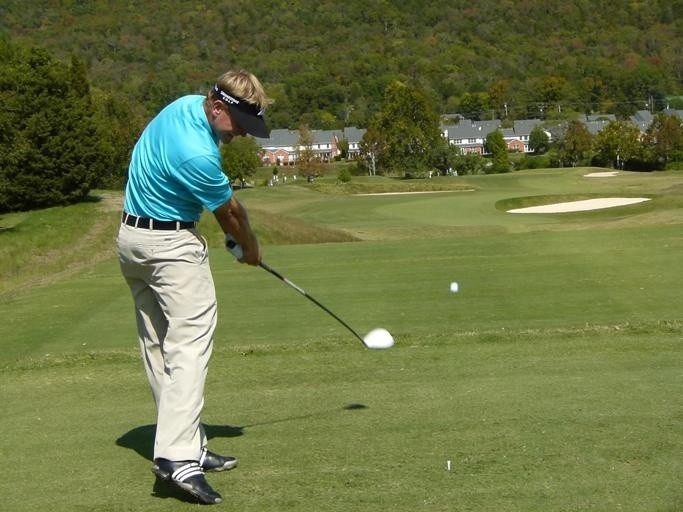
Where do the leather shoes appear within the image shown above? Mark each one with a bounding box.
[197,446,237,473]
[151,457,223,505]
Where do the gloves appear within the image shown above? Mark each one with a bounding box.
[222,234,264,267]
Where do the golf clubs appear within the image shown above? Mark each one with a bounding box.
[225,237,394,350]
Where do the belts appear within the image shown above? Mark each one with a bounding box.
[120,212,195,232]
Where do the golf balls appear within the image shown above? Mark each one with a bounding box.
[451,281,459,290]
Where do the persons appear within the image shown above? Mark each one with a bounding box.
[117,69,262,506]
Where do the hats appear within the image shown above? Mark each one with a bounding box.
[212,83,272,140]
